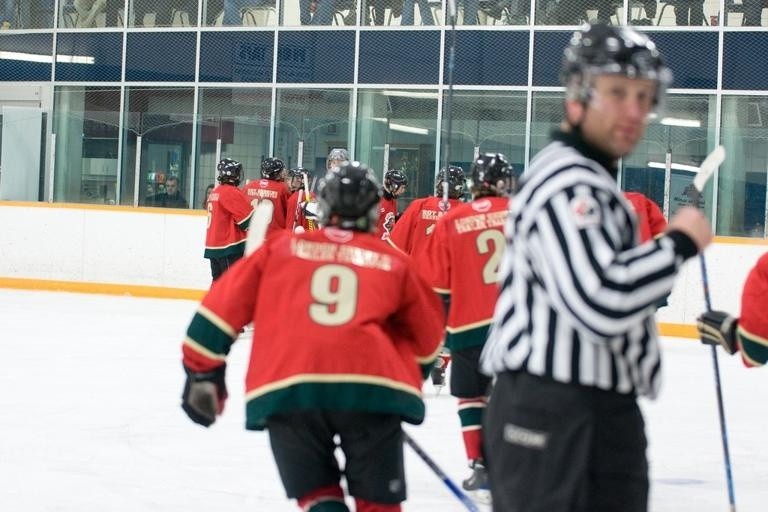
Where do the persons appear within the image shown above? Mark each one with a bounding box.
[420,150,513,490]
[479,19,713,512]
[388,164,467,386]
[240,149,352,258]
[377,171,406,241]
[695,250,767,369]
[202,184,214,209]
[0,0,767,28]
[178,160,445,512]
[145,175,189,207]
[623,191,666,244]
[203,160,255,334]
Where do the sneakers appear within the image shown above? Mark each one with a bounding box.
[432,368,446,384]
[463,467,488,489]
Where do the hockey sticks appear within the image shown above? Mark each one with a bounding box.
[241,199,481,512]
[690,145,737,512]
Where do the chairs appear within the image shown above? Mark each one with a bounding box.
[61,4,710,27]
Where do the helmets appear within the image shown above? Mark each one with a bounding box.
[436,154,515,198]
[563,24,670,83]
[218,157,308,191]
[316,148,408,218]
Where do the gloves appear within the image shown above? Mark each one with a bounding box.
[697,311,738,354]
[182,362,227,426]
